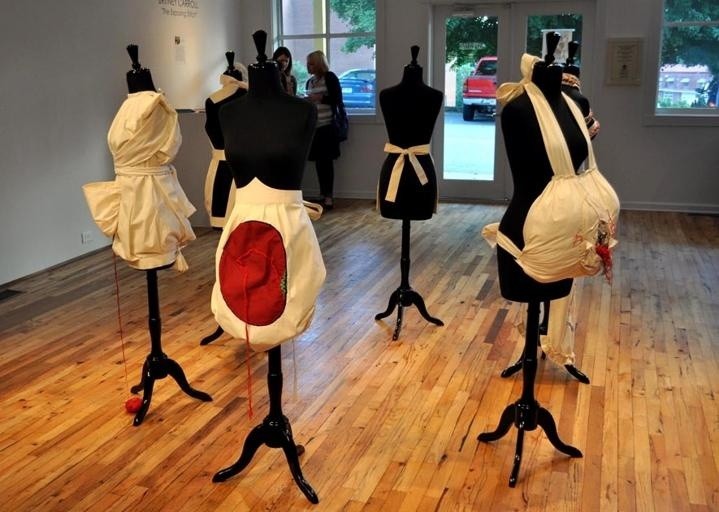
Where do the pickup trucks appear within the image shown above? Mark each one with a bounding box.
[462,58,497,120]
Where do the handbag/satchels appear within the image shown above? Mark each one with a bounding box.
[333,104,348,141]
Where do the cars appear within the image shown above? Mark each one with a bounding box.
[338,70,375,115]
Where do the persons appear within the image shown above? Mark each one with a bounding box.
[272,47,296,97]
[115,27,602,506]
[306,51,346,212]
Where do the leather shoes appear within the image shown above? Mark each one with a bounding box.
[325,200,334,210]
